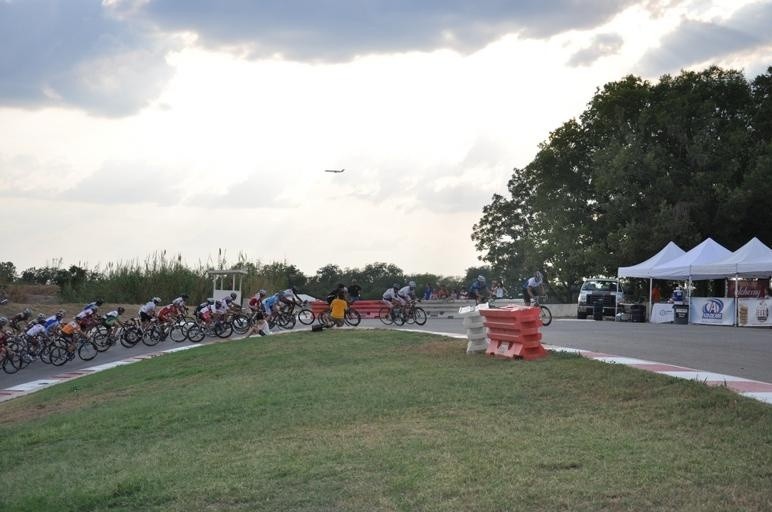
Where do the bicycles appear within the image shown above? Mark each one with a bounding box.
[468,293,497,308]
[380,299,417,325]
[391,299,427,326]
[518,292,553,326]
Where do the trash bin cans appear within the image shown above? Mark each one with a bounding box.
[593,300,604,321]
[631,305,646,322]
[672,304,689,324]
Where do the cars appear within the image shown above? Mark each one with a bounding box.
[578,279,626,319]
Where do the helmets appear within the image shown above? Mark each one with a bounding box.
[408,281,416,290]
[533,271,544,281]
[207,293,237,307]
[279,290,285,296]
[292,285,300,292]
[478,275,485,282]
[153,293,189,308]
[392,282,401,290]
[260,290,266,296]
[0,298,125,327]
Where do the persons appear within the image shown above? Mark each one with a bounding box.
[326,279,362,326]
[0,286,303,356]
[652,283,661,303]
[672,286,683,302]
[382,274,546,311]
